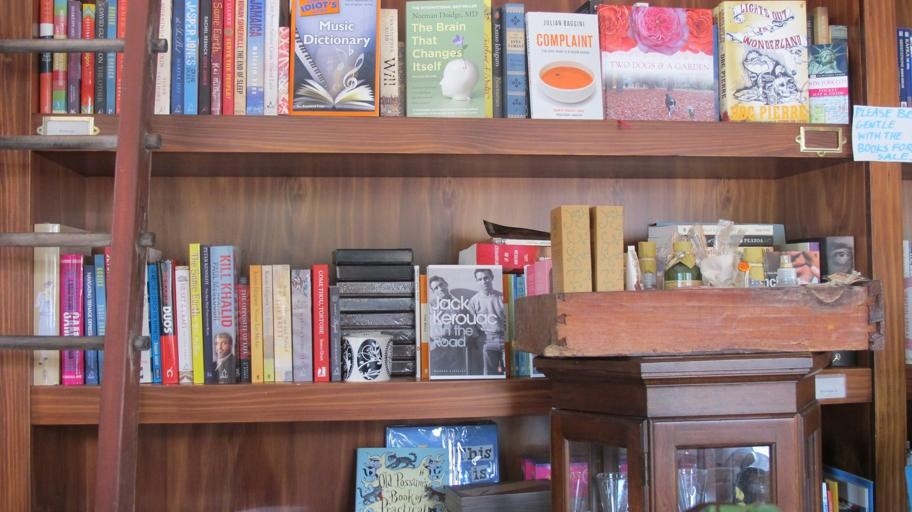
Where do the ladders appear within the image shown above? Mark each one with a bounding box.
[0,0,167,512]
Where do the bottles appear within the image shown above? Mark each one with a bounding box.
[664,235,702,287]
[732,261,749,287]
[638,241,656,288]
[776,254,797,287]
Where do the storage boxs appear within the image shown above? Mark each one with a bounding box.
[508,280,887,358]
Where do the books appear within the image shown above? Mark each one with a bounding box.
[406,1,850,124]
[820,462,874,512]
[898,28,911,107]
[34,0,405,117]
[458,223,854,378]
[34,223,507,385]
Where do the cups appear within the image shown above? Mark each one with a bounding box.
[343,333,393,384]
[677,466,708,508]
[595,471,626,512]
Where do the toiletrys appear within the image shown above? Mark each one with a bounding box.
[624,244,643,291]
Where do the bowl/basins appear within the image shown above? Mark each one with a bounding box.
[536,59,597,105]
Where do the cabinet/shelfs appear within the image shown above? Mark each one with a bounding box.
[529,348,839,512]
[0,0,887,512]
[887,1,912,511]
